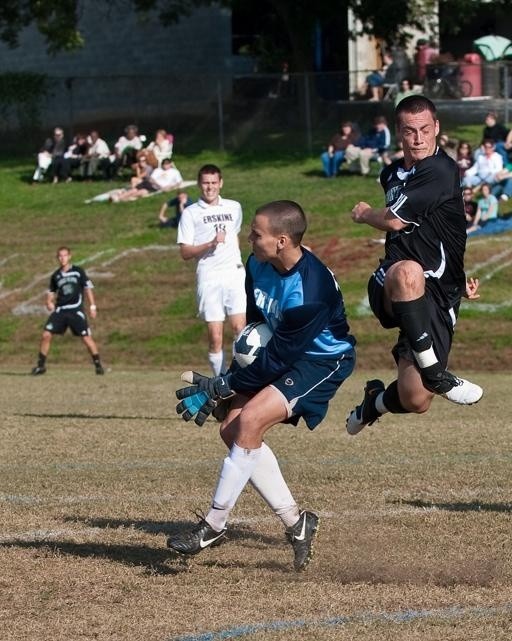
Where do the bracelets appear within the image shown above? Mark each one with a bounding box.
[90,304,96,311]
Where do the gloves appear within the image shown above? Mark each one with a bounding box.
[175,359,244,425]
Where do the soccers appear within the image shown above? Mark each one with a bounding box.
[233,321,273,371]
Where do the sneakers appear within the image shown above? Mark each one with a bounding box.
[422,368,483,405]
[285,509,320,573]
[167,506,228,554]
[95,366,103,375]
[32,367,45,375]
[346,379,385,435]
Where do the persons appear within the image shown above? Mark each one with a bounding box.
[343,93,485,436]
[176,163,248,378]
[165,199,358,574]
[27,121,184,204]
[30,245,104,375]
[319,33,512,235]
[157,190,193,229]
